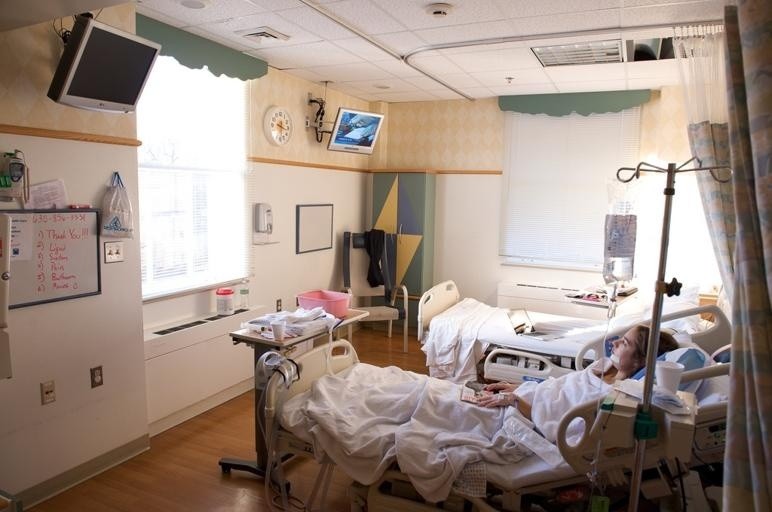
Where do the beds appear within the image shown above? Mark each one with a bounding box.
[261,342,731,512]
[415,280,730,387]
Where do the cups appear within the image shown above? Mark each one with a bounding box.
[654,360,685,394]
[270,320,286,341]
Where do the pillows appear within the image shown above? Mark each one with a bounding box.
[609,302,699,335]
[629,349,712,395]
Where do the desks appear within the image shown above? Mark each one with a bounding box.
[572,285,637,309]
[219,308,370,493]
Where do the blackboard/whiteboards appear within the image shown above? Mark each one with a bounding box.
[0,208,102,311]
[296,204,334,254]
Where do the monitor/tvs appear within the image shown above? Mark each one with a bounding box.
[327,107,384,155]
[47,14,162,114]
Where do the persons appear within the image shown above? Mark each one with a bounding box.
[344,114,379,139]
[476,325,680,443]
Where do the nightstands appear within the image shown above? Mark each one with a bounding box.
[699,294,718,323]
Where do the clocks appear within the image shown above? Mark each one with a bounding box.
[264,105,292,147]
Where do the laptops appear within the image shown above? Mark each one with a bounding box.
[506,307,564,342]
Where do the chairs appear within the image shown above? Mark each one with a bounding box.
[336,232,408,352]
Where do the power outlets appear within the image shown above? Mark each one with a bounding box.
[104,241,124,263]
[90,366,103,388]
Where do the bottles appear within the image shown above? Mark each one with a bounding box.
[216,288,235,316]
[347,288,354,309]
[239,279,249,310]
